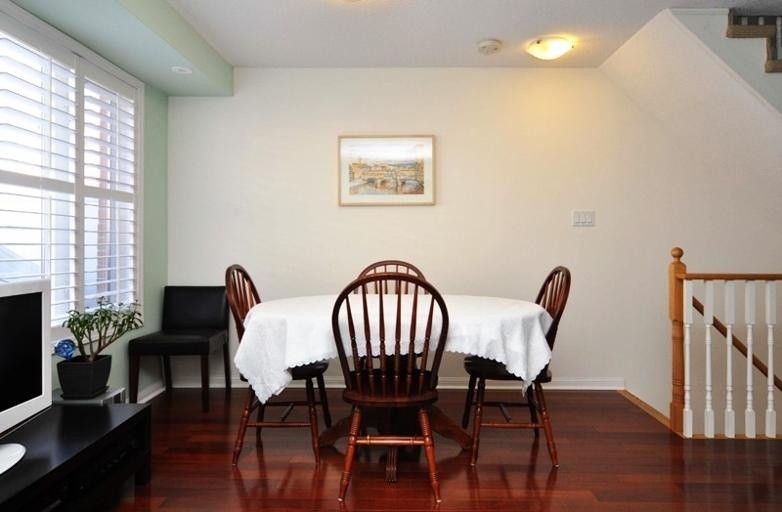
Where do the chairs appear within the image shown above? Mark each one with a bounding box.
[353,260,429,295]
[224,264,331,466]
[331,272,449,503]
[127,286,233,415]
[462,265,570,466]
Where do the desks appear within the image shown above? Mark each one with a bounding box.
[0,402,151,510]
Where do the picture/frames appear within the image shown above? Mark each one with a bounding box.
[334,131,436,208]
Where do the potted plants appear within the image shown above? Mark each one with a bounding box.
[50,298,143,400]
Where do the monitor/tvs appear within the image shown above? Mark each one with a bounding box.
[0,276,52,475]
